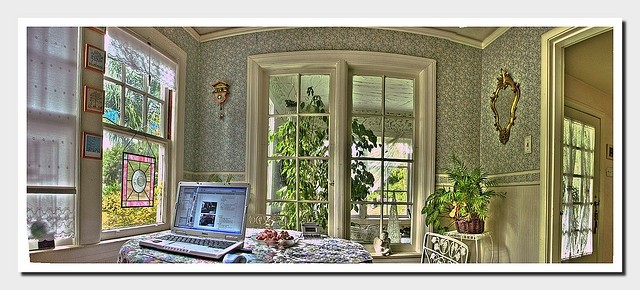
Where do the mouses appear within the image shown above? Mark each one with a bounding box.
[221,253,246,263]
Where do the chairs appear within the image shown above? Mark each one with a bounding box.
[420,231,471,263]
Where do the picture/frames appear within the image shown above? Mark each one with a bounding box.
[88,27,107,36]
[81,131,103,159]
[84,42,106,73]
[83,84,105,115]
[606,143,613,159]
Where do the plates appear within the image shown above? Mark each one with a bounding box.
[251,233,300,247]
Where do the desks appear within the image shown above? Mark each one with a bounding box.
[122,227,373,263]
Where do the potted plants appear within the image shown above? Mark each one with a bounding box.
[421,153,507,236]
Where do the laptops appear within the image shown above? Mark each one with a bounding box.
[139,181,252,259]
